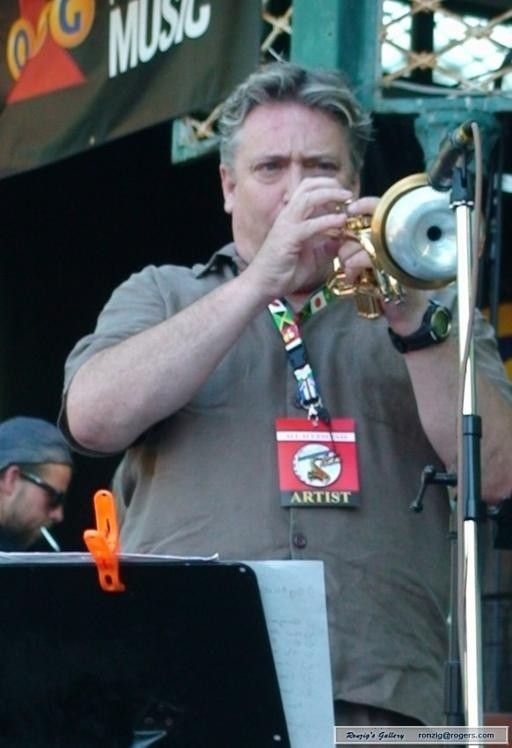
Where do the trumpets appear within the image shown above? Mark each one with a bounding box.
[320,174,486,319]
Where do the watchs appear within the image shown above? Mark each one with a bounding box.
[387,297,453,354]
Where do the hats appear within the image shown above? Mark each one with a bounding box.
[0,414,74,472]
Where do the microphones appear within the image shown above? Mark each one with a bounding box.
[426,121,473,190]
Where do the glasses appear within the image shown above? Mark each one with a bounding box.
[21,474,68,510]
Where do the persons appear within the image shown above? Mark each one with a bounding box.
[56,63,511,748]
[1,415,90,552]
